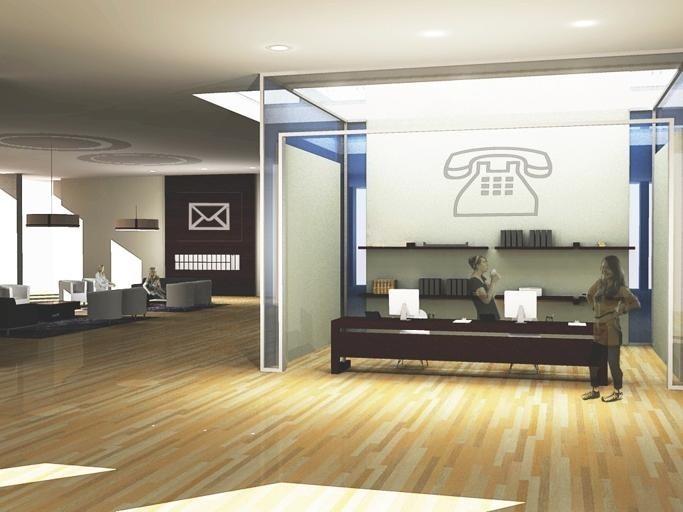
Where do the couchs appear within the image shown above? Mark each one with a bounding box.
[130,277,193,306]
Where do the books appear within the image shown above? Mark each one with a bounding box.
[418,278,472,297]
[371,278,397,296]
[499,229,552,248]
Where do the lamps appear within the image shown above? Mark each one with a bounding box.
[25,145,80,228]
[112,205,160,233]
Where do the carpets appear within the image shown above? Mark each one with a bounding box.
[5,315,160,338]
[145,301,230,313]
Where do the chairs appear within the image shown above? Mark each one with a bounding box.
[58,277,114,307]
[166,279,213,311]
[85,287,149,325]
[509,304,543,373]
[0,298,38,335]
[397,308,428,369]
[0,284,30,306]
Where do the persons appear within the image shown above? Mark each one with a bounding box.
[93,263,116,291]
[467,255,502,320]
[580,255,642,403]
[141,267,165,300]
